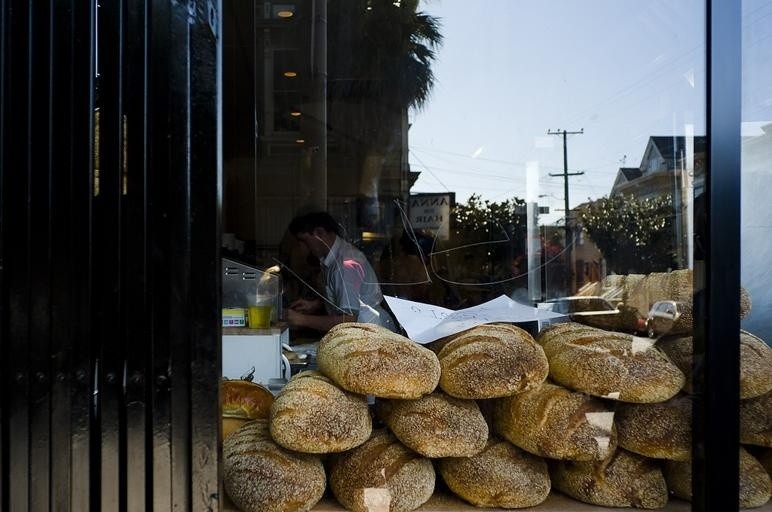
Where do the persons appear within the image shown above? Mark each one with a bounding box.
[278,209,402,339]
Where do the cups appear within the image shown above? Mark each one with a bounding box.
[246,293,273,330]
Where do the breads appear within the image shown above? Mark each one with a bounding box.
[222,268,772,511]
